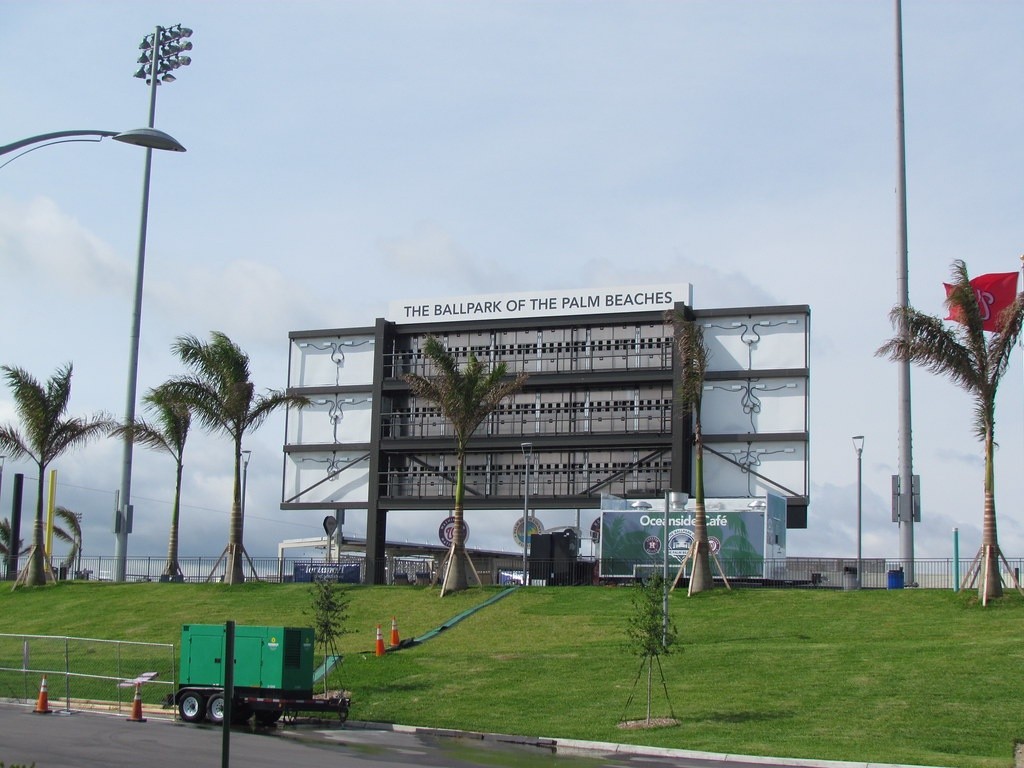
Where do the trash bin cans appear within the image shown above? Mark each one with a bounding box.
[887,569,904,589]
[843,566,858,589]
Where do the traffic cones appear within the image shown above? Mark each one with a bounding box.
[127,684,147,722]
[33,675,53,713]
[388,615,399,647]
[375,623,386,657]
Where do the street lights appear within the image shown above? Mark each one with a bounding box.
[852,434,866,591]
[521,441,533,588]
[112,24,194,587]
[0,454,6,498]
[240,448,252,547]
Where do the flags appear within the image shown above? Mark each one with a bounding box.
[942,271,1020,333]
